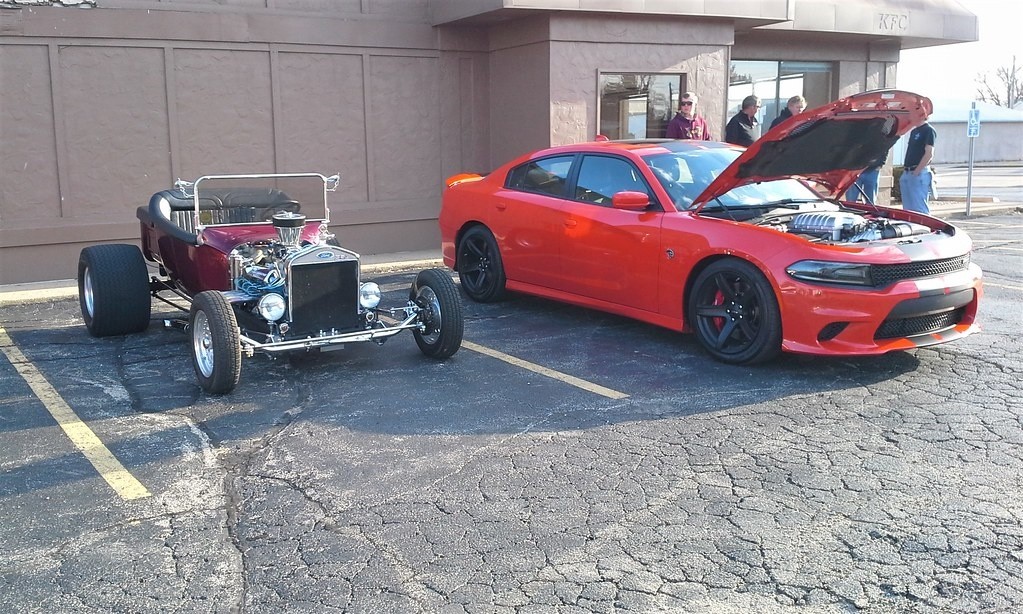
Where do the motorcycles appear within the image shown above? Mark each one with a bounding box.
[77,172,463,395]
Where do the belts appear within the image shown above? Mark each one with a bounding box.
[904,166,916,170]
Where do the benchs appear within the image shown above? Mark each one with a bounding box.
[150,187,304,234]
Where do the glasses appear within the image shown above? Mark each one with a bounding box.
[679,100,693,106]
[755,105,759,108]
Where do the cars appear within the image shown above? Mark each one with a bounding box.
[438,88,983,366]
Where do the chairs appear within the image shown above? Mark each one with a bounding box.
[653,158,680,182]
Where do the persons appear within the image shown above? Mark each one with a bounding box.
[846,148,889,203]
[770,96,807,129]
[898,116,937,215]
[666,92,711,140]
[726,95,761,147]
